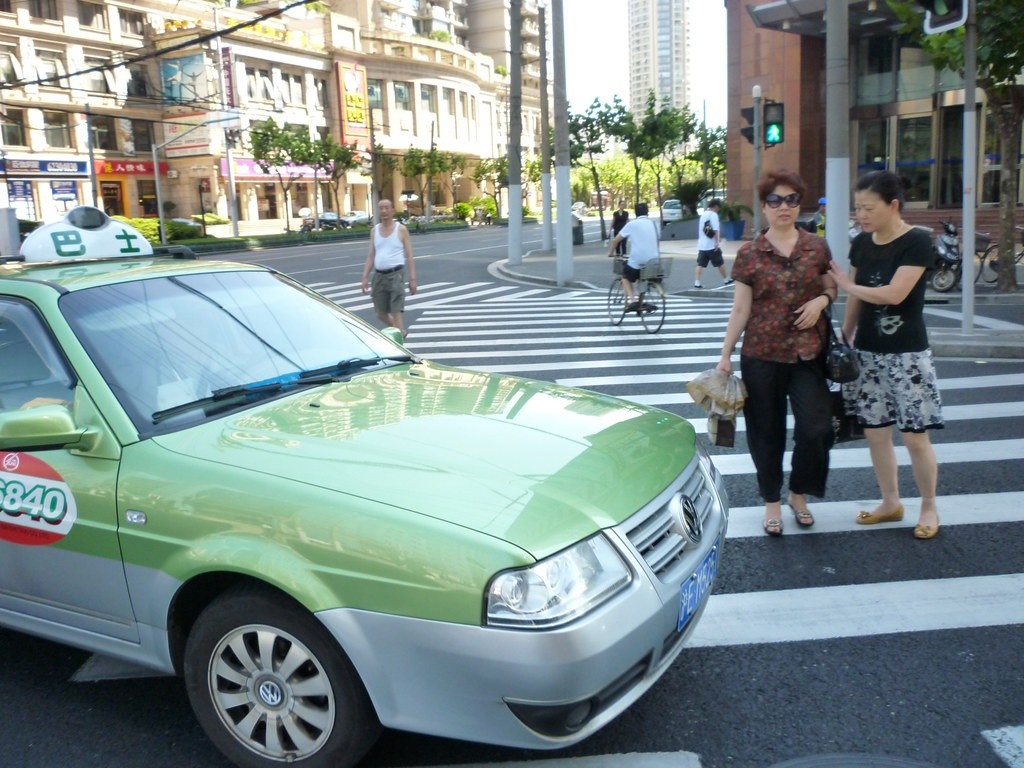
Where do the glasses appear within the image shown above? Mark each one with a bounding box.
[765,193,802,208]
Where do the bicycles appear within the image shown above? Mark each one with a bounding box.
[954,231,992,293]
[981,225,1024,284]
[608,254,672,333]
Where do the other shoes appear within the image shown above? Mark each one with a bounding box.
[637,311,640,317]
[694,285,705,289]
[724,279,735,284]
[856,505,904,524]
[913,514,938,538]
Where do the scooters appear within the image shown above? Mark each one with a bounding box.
[925,219,962,293]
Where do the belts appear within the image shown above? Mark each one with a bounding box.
[376,265,403,274]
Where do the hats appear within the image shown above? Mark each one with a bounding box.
[818,198,826,205]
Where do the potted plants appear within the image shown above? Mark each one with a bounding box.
[710,200,753,241]
[164,201,176,216]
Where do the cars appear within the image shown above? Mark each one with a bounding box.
[1,245,728,768]
[660,199,690,226]
[340,211,368,228]
[303,213,349,231]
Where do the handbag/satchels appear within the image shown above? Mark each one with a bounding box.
[648,268,664,288]
[826,385,866,444]
[703,221,715,238]
[604,236,611,248]
[814,293,860,383]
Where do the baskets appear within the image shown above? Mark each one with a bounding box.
[613,259,625,274]
[975,235,992,252]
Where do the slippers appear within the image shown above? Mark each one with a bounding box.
[789,502,814,527]
[763,519,784,535]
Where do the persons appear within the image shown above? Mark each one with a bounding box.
[477,209,483,226]
[695,199,735,288]
[813,197,825,237]
[716,167,838,538]
[828,170,944,538]
[362,200,416,336]
[609,203,660,316]
[608,201,629,255]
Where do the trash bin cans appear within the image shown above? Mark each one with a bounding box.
[572,220,583,245]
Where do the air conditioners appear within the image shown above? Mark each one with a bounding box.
[168,171,179,179]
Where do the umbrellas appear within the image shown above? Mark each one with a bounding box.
[399,194,419,202]
[298,208,311,216]
[686,368,747,418]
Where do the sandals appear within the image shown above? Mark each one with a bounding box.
[624,302,640,312]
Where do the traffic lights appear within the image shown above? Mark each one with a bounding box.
[912,0,969,35]
[739,107,753,145]
[763,103,784,143]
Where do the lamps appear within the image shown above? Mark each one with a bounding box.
[783,20,791,31]
[868,0,877,13]
